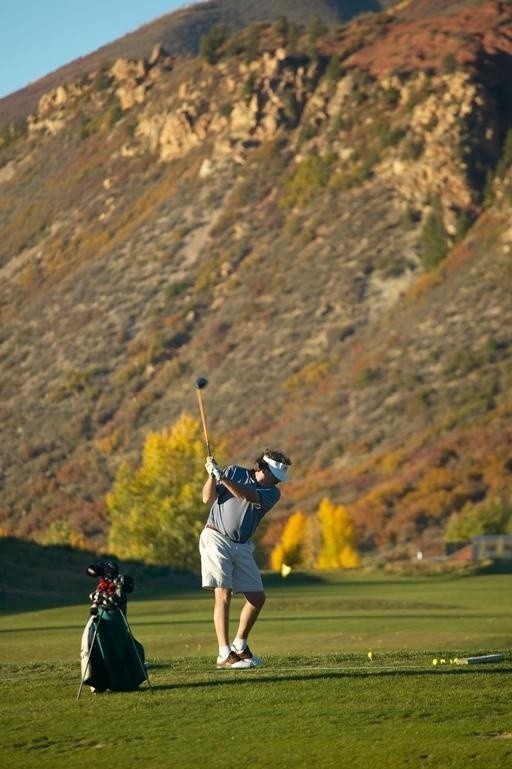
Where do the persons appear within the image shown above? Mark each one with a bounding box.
[195,446,293,668]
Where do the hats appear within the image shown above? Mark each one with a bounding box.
[263,455,290,482]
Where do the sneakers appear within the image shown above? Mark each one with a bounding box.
[216,644,260,669]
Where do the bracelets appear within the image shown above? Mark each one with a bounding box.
[211,464,226,481]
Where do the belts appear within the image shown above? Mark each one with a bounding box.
[207,525,222,535]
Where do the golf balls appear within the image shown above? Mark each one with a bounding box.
[433,657,459,665]
[368,651,375,657]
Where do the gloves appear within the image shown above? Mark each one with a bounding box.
[204,462,225,482]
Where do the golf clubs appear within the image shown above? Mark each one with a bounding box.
[86,561,133,594]
[195,377,211,458]
